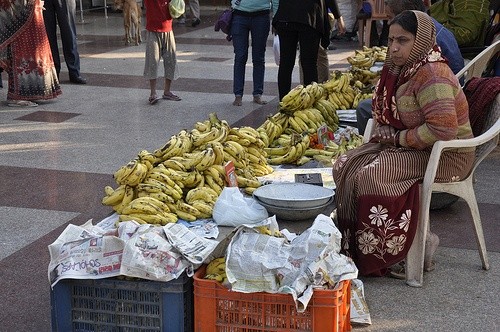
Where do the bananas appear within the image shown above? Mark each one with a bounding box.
[101,112,272,228]
[255,46,388,166]
[202,225,282,280]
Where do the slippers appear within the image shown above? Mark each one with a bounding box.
[390,266,405,279]
[7,100,38,107]
[162,91,182,101]
[149,95,160,104]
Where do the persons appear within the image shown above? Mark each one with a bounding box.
[143,0,182,103]
[333,11,476,277]
[176,0,201,26]
[356,0,465,137]
[0,0,87,106]
[228,0,344,106]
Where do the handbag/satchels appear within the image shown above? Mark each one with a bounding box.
[215,9,232,35]
[273,35,280,65]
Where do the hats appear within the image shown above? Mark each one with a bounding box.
[168,0,185,18]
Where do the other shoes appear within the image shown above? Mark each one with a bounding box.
[69,76,88,84]
